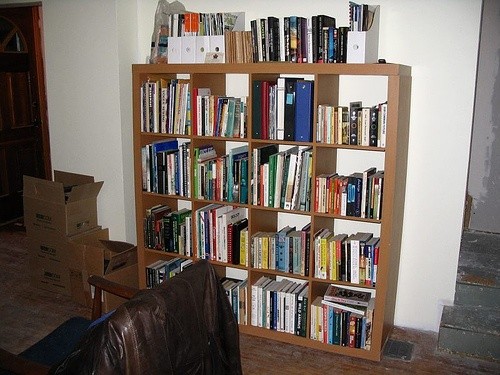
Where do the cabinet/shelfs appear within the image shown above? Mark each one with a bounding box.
[130,63,413,362]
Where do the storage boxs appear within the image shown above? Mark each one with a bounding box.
[23,169,140,321]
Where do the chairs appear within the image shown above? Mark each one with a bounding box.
[0,259,209,375]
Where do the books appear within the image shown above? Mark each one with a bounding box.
[140,2,387,352]
